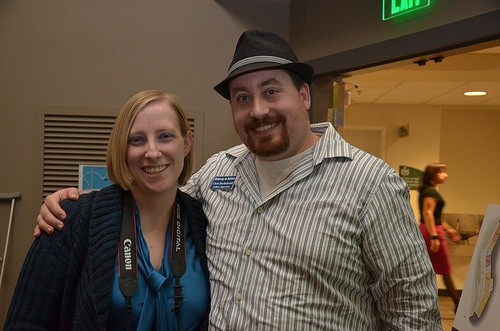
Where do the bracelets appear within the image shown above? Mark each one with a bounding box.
[431,236,439,240]
[444,226,453,231]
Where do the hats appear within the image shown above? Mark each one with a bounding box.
[213,30,315,100]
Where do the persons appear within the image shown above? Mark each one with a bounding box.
[419,163,462,314]
[4,90,211,331]
[34,30,443,331]
